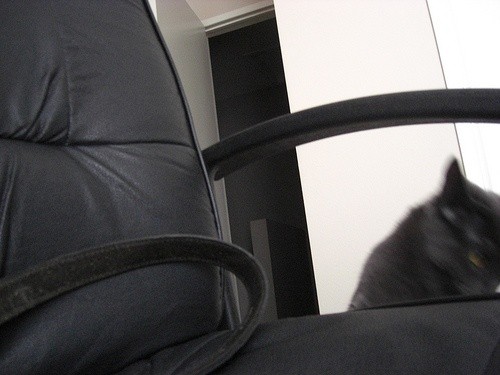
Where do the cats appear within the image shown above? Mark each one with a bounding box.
[348,156,500,311]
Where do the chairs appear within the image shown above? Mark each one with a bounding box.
[0,0,499,375]
